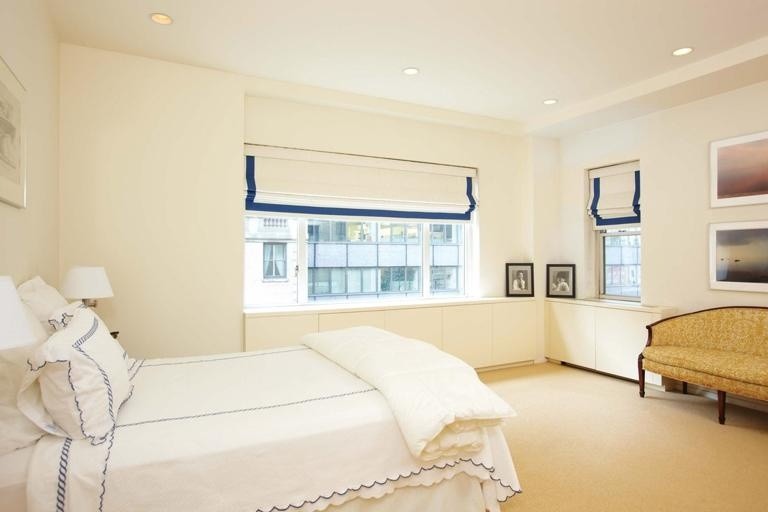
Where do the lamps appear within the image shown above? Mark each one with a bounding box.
[60,266,114,312]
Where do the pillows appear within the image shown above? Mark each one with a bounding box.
[0,272,137,454]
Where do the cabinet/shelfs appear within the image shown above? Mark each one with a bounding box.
[544,301,677,392]
[245,298,540,377]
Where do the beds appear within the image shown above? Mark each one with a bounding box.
[0,324,498,512]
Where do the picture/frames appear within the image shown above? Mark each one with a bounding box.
[546,264,576,298]
[706,129,768,294]
[505,262,534,297]
[0,55,30,213]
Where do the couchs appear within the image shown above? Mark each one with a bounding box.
[637,305,767,425]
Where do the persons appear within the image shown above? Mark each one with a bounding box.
[513,271,525,290]
[551,271,569,292]
